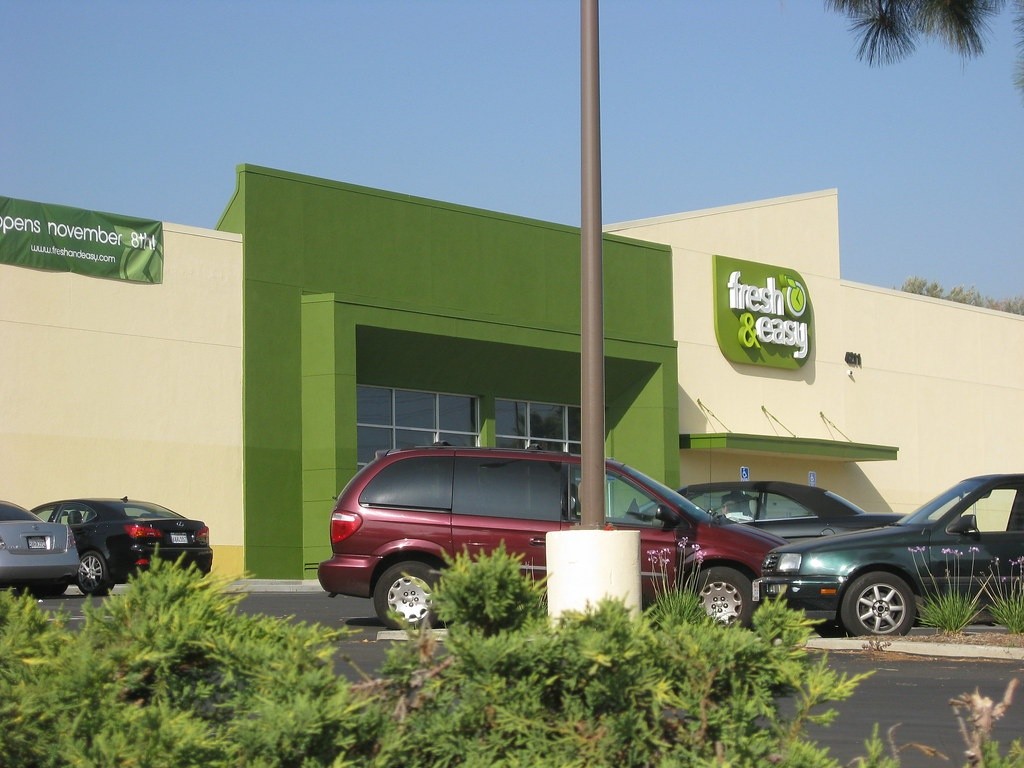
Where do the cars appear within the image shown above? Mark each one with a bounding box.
[631,473,1024,637]
[1,495,212,596]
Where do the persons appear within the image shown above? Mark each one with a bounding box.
[720,494,756,523]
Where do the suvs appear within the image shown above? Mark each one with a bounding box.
[315,440,794,635]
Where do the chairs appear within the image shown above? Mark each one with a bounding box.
[67,511,82,524]
